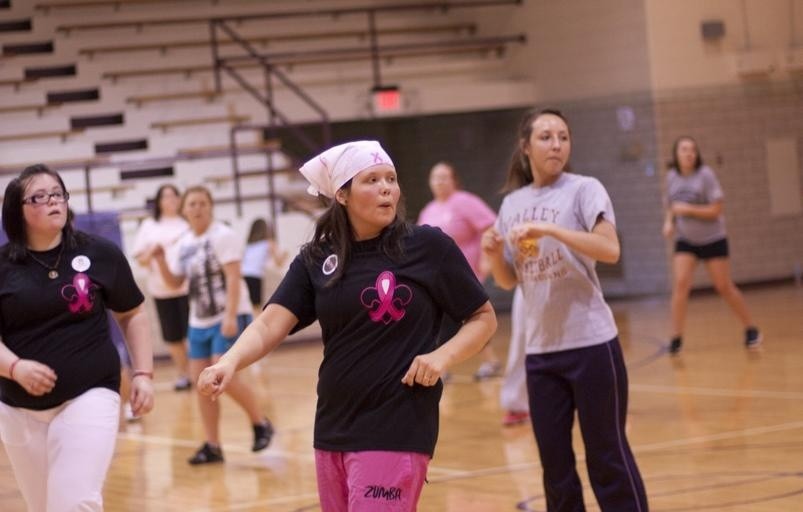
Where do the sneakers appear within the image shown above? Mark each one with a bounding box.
[501,411,528,426]
[743,324,764,349]
[174,376,191,391]
[189,441,224,465]
[473,359,505,380]
[669,335,685,355]
[252,415,275,452]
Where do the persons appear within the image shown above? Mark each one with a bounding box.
[413,160,501,381]
[239,212,289,312]
[129,183,194,391]
[661,133,766,355]
[139,183,275,466]
[195,138,499,512]
[0,162,156,511]
[480,105,650,511]
[500,284,530,426]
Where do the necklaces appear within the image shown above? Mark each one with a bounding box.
[26,240,65,281]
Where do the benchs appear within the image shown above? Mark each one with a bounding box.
[0,1,509,358]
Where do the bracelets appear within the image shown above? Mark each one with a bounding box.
[8,356,23,382]
[131,370,154,381]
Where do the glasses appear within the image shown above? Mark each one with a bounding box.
[21,191,70,206]
